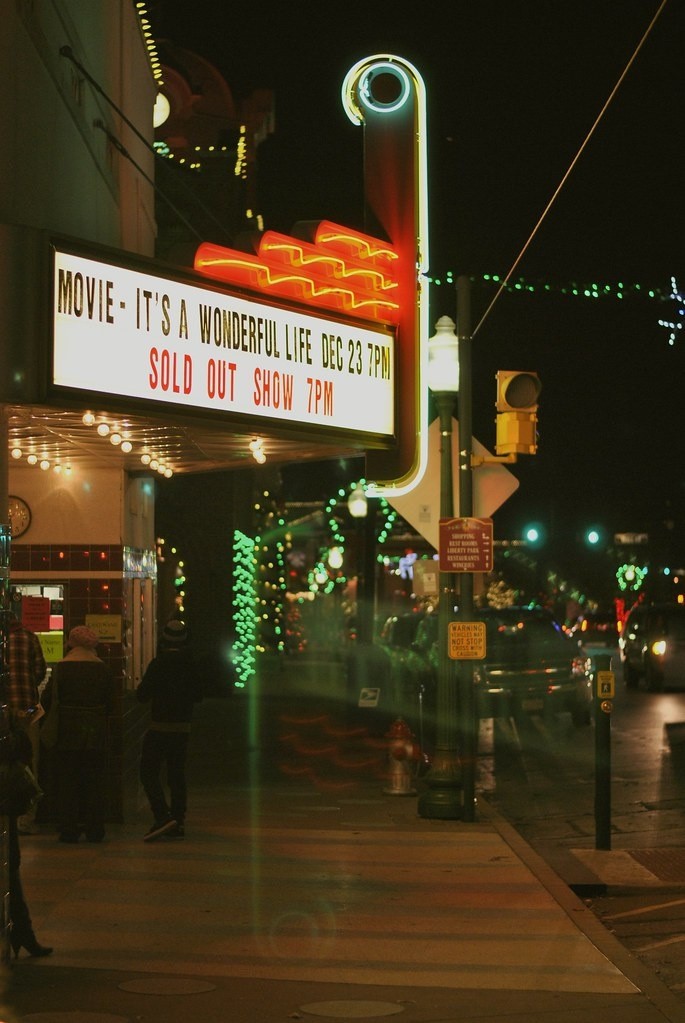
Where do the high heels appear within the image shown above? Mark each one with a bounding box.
[9,930,54,959]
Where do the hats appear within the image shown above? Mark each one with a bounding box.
[0,592,21,621]
[69,626,99,648]
[156,620,192,652]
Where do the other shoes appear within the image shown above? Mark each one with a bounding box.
[157,825,185,841]
[143,812,177,841]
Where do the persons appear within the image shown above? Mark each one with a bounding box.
[0,609,47,837]
[0,709,54,957]
[135,620,205,844]
[41,626,115,844]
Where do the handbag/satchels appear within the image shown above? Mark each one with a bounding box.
[0,700,44,814]
[40,667,58,750]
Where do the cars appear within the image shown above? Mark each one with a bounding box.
[620,599,685,693]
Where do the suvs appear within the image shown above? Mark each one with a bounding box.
[473,606,594,730]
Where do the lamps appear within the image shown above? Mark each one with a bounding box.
[141,454,150,465]
[110,433,122,446]
[64,467,72,475]
[28,454,38,465]
[248,438,267,464]
[53,464,62,474]
[150,459,159,470]
[82,414,95,426]
[120,440,133,453]
[164,468,173,478]
[11,449,22,459]
[158,464,166,474]
[97,423,110,437]
[40,460,50,471]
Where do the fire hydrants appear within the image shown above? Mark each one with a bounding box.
[369,721,431,796]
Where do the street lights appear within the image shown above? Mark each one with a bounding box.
[416,315,480,819]
[345,480,374,725]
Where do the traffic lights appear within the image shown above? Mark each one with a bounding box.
[496,371,542,412]
